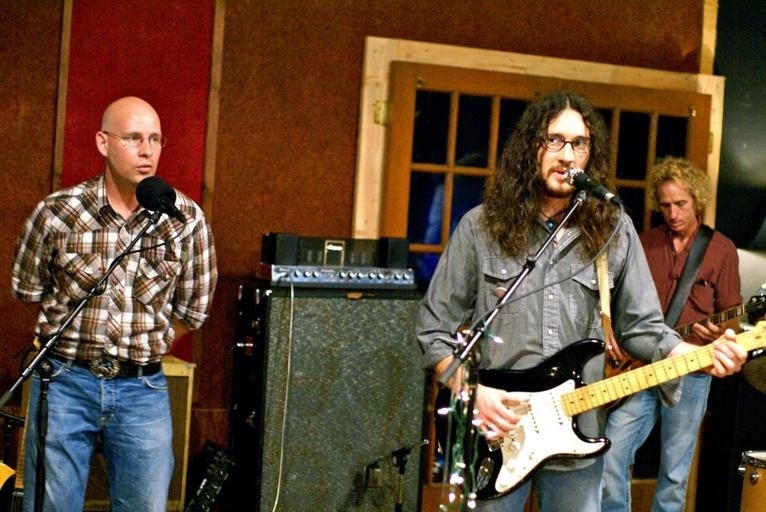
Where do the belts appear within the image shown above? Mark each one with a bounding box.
[47,355,163,380]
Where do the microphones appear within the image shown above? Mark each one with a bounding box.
[565,168,623,204]
[135,175,188,223]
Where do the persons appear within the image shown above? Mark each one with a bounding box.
[413,89,748,512]
[10,96,220,511]
[598,156,743,512]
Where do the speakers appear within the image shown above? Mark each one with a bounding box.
[229,285,428,510]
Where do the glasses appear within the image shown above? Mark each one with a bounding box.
[542,134,592,153]
[103,131,167,151]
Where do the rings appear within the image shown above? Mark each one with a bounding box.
[489,422,496,431]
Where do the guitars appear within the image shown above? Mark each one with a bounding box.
[435,319,766,500]
[600,283,766,412]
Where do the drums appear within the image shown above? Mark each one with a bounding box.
[737,449,766,511]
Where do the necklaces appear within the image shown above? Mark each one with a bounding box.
[537,209,568,230]
